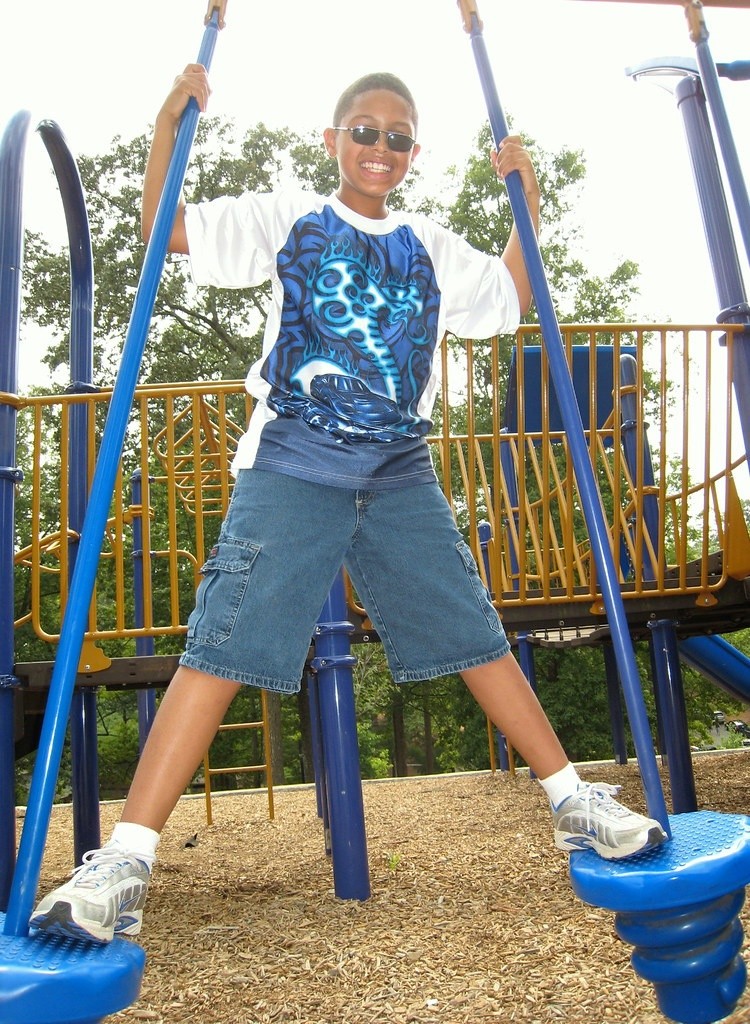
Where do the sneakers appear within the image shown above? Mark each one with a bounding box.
[27,839,149,944]
[551,782,668,861]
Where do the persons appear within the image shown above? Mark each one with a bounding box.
[30,62,672,944]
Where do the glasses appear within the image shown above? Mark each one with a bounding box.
[334,126,416,153]
